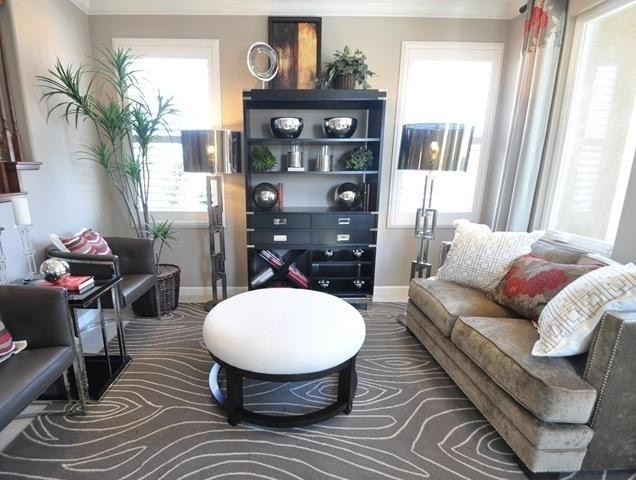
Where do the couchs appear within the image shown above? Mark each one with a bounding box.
[407,235,636,478]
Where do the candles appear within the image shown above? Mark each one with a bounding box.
[10,197,32,228]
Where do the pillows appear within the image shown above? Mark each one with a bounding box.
[0,317,19,362]
[435,219,546,294]
[47,227,113,258]
[530,263,636,357]
[494,254,605,320]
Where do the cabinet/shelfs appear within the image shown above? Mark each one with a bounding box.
[242,89,386,312]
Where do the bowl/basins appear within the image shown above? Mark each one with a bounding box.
[321,117,358,138]
[270,117,303,139]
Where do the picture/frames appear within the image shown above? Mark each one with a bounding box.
[267,15,324,91]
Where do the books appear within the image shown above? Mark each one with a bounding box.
[250,266,273,289]
[67,282,94,295]
[287,264,310,289]
[260,249,285,268]
[31,275,95,290]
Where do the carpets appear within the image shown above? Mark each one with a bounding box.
[0,302,549,479]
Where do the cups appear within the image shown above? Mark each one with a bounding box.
[286,144,304,168]
[316,144,333,172]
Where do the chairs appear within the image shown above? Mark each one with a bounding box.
[0,287,85,435]
[44,237,161,361]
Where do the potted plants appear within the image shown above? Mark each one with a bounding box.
[315,46,379,90]
[34,42,179,317]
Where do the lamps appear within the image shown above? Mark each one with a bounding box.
[183,129,242,311]
[397,123,474,280]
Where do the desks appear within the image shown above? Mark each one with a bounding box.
[11,274,136,407]
